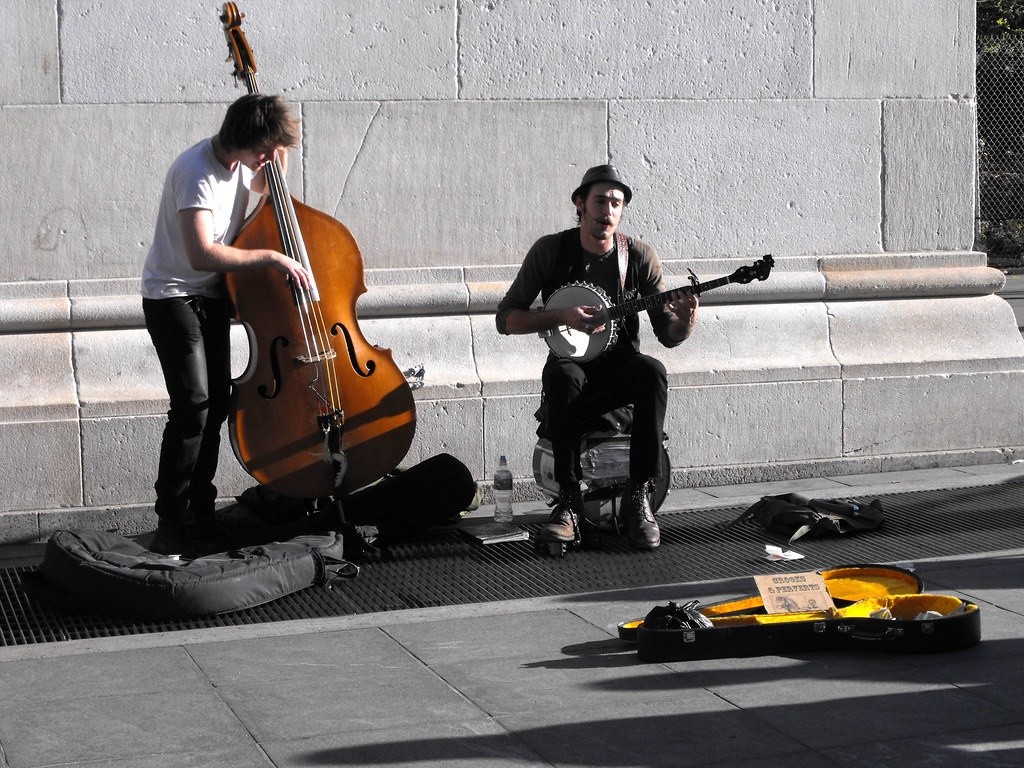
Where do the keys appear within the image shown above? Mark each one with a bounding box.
[184,295,206,318]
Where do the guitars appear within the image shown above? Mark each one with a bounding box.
[540,254,775,364]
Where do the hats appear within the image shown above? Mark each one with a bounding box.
[571,165,632,206]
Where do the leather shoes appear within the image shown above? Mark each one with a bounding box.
[149,507,230,555]
[619,477,660,548]
[535,487,585,542]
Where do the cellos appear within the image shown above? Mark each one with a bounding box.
[220,3,417,523]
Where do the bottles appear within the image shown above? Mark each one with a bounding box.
[494,455,514,523]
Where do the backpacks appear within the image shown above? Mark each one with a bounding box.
[716,492,884,546]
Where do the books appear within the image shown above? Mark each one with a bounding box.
[456,517,530,545]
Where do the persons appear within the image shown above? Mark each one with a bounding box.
[495,165,700,548]
[141,93,315,558]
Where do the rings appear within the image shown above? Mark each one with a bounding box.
[584,324,591,332]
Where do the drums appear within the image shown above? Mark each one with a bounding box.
[533,406,671,529]
